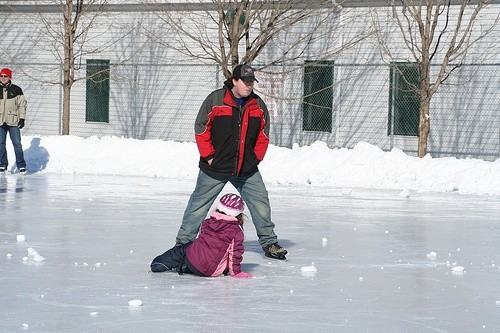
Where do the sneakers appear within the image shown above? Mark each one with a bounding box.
[261,243,287,260]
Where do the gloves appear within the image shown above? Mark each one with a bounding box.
[18,119,25,128]
[234,271,253,278]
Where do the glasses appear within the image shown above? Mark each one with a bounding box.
[1,75,9,77]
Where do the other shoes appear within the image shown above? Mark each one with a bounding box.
[20,168,27,174]
[0,166,8,174]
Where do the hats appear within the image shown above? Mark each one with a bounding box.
[215,192,245,216]
[0,68,12,77]
[233,64,258,83]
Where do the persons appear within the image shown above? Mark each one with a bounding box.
[0,68,27,173]
[174,64,288,260]
[151,193,252,278]
